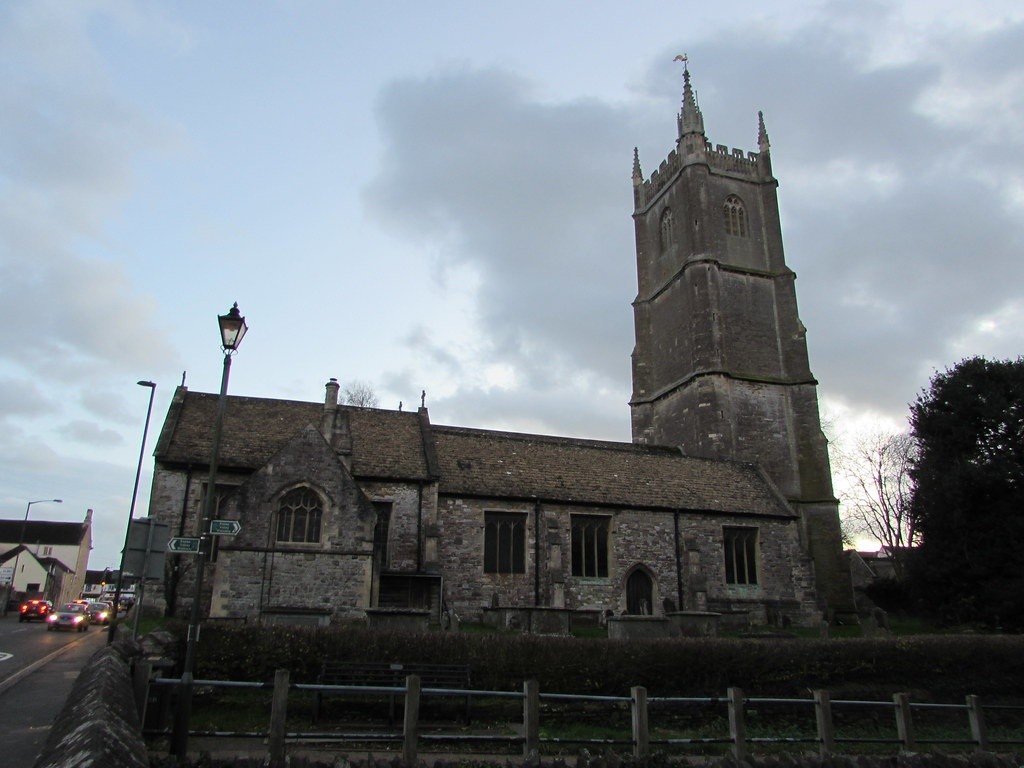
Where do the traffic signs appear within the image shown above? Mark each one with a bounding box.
[209,518,241,536]
[167,536,205,555]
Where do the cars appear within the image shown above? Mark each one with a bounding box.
[72,596,131,613]
[49,602,90,633]
[85,603,112,625]
[19,600,53,622]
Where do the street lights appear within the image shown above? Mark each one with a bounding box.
[170,299,252,765]
[105,379,158,647]
[3,499,63,616]
